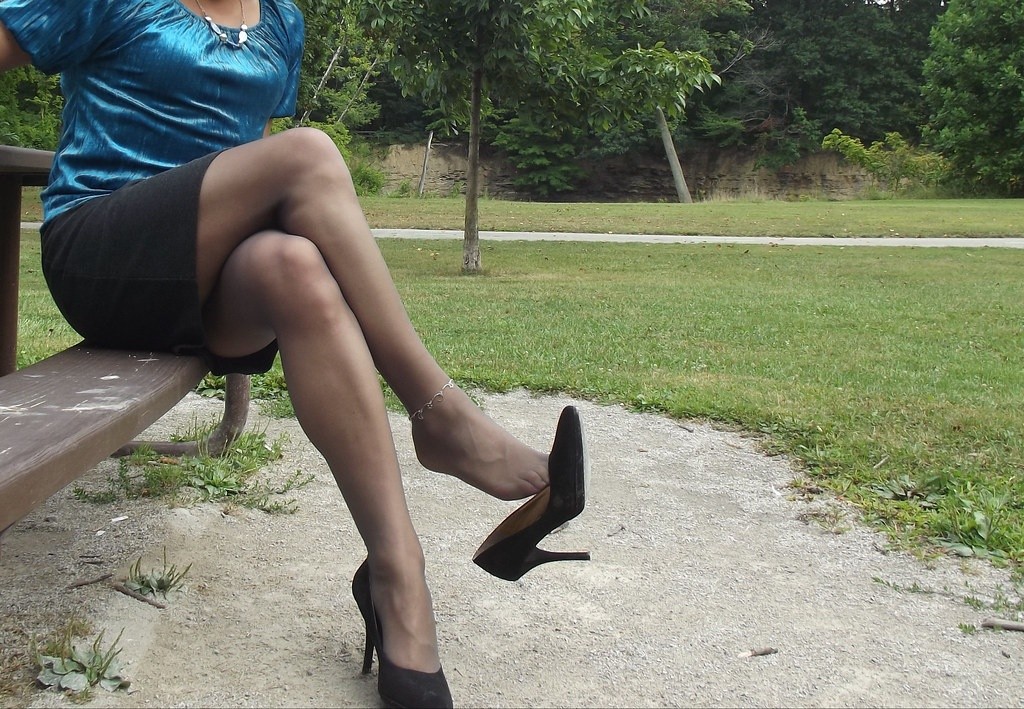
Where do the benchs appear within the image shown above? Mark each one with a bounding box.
[0,146,258,534]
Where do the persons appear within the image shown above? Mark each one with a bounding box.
[0,0,591,709]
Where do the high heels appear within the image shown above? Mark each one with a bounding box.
[352,557,453,709]
[472,406,590,581]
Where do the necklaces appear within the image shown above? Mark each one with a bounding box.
[197,0,247,47]
